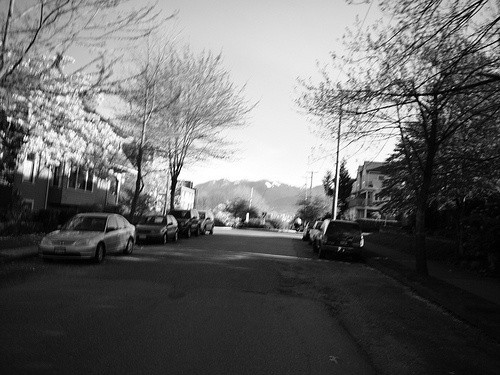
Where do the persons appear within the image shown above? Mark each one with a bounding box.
[294,216,302,229]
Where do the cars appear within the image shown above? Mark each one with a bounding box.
[35,211,137,264]
[197,212,215,237]
[308,219,325,245]
[131,214,179,247]
[167,209,202,241]
[313,219,365,262]
[303,221,314,240]
